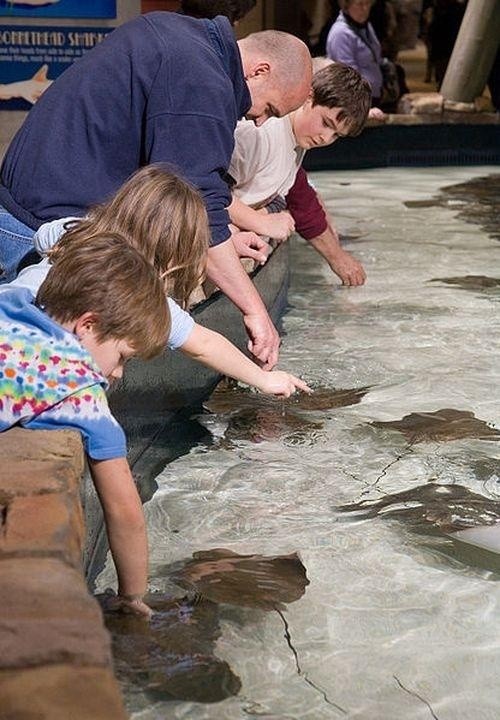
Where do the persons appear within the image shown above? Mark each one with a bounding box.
[0,162,314,401]
[225,64,373,243]
[285,57,366,287]
[329,0,382,107]
[0,12,311,373]
[490,54,499,111]
[0,232,171,617]
[431,0,465,91]
[418,1,433,84]
